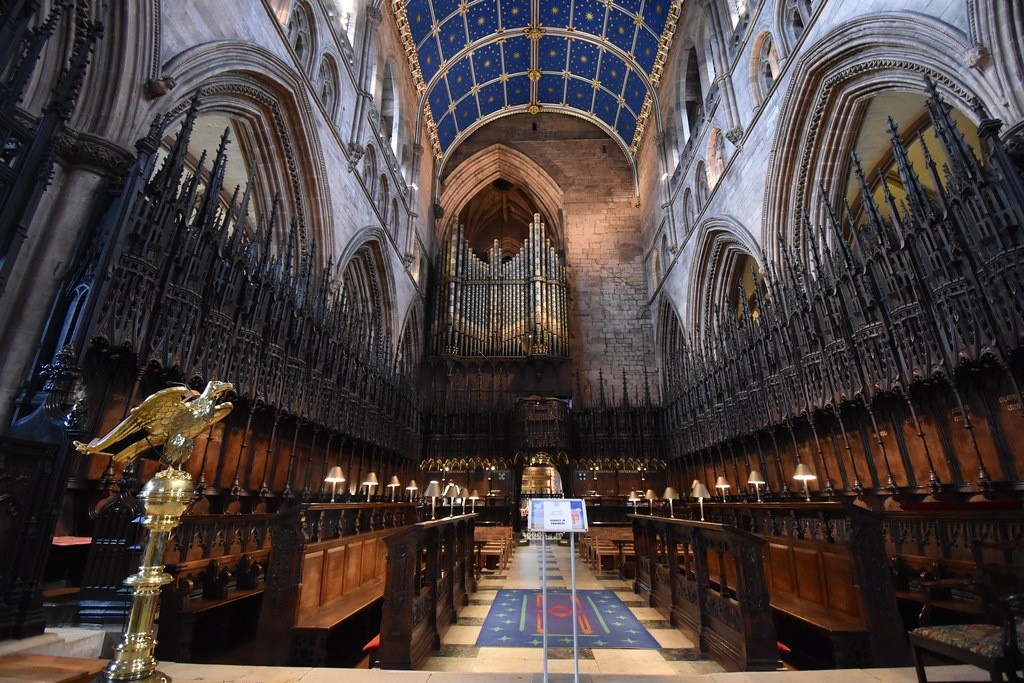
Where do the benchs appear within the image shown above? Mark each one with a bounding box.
[80,484,512,671]
[580,501,1023,670]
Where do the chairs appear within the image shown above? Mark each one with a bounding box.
[908,540,1024,683]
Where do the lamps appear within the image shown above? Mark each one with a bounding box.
[629,463,816,522]
[326,468,478,519]
